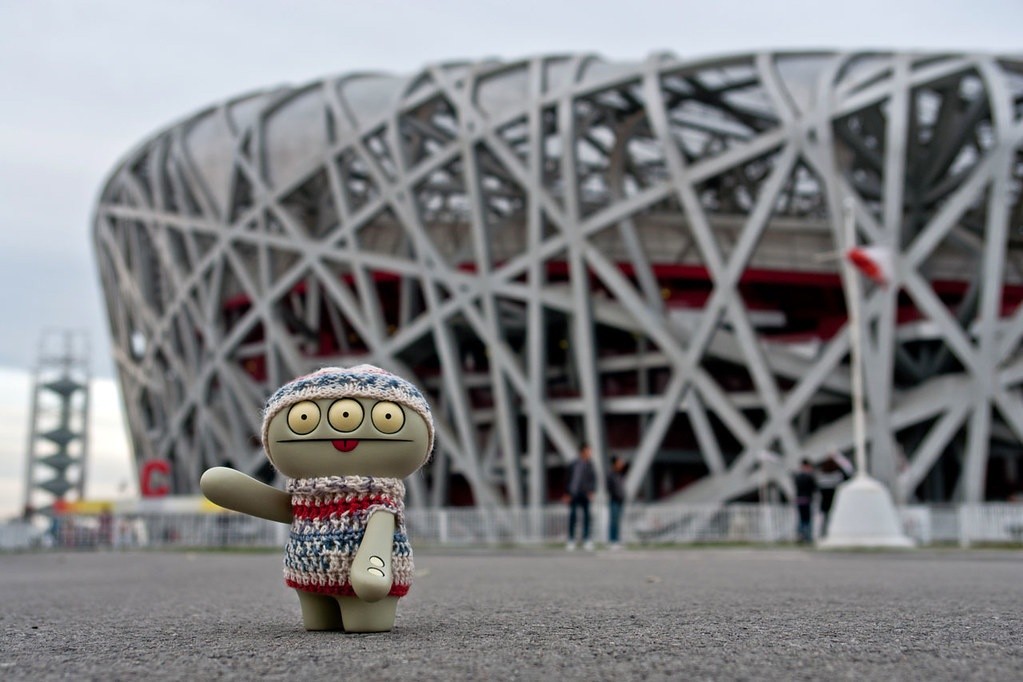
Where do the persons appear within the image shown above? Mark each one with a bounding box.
[795,458,842,546]
[605,455,633,548]
[562,443,597,550]
